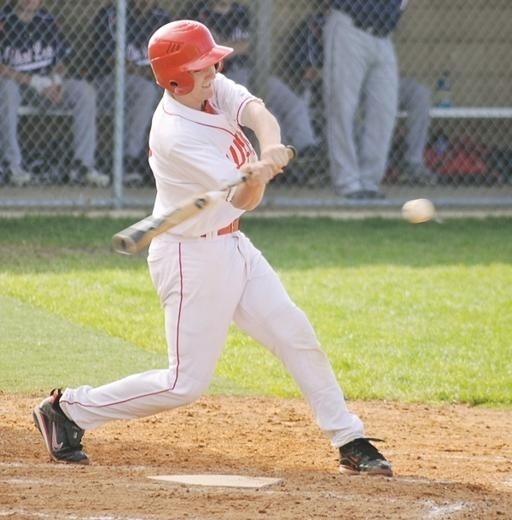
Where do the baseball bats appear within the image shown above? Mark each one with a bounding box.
[112,146,296,255]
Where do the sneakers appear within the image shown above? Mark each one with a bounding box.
[121,158,144,186]
[7,163,31,185]
[67,168,111,187]
[396,164,439,186]
[361,179,384,200]
[339,438,393,478]
[333,179,364,200]
[32,388,90,465]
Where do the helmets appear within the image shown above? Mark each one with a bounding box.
[148,20,234,97]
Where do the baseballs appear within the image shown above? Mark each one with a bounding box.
[400,198,434,222]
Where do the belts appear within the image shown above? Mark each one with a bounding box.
[200,218,239,237]
[352,19,389,38]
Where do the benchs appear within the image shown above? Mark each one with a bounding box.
[18,102,512,120]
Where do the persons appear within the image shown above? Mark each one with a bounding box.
[0,1,438,199]
[32,19,394,477]
